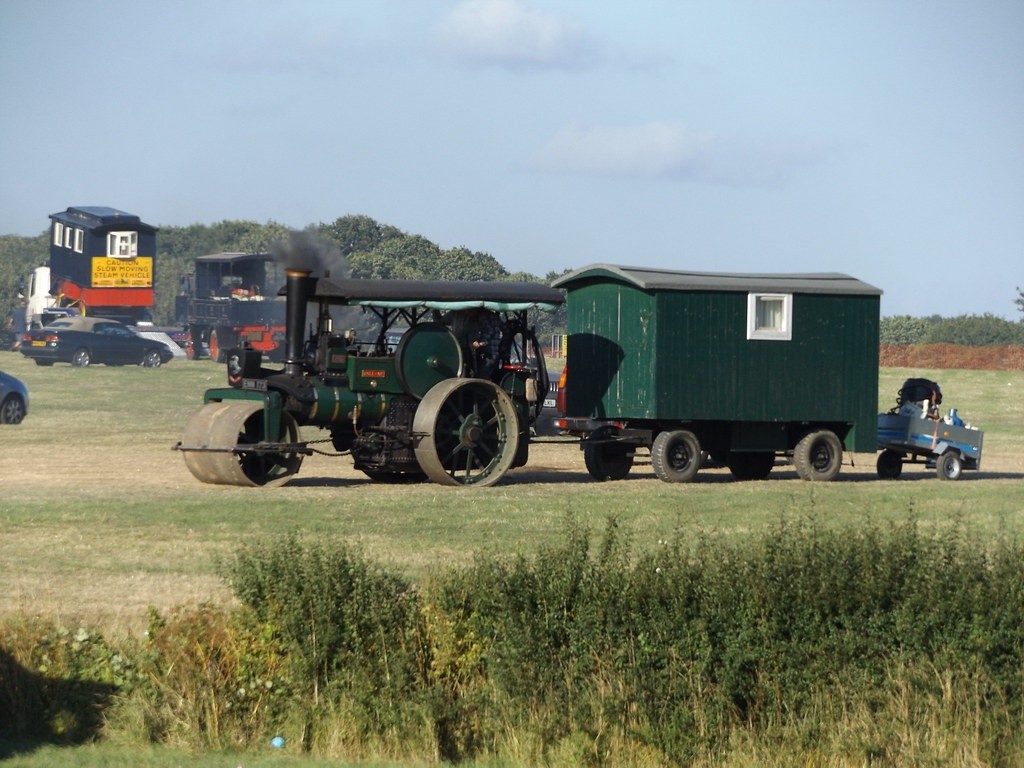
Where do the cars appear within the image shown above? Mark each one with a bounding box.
[24,314,174,368]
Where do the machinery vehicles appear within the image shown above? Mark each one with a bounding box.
[180,269,986,486]
[31,205,160,328]
[174,248,288,360]
